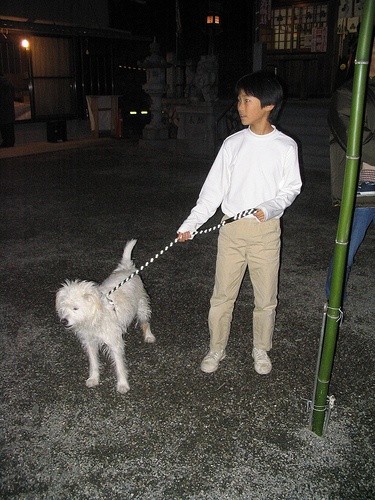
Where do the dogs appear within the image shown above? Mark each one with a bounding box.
[55,238,158,395]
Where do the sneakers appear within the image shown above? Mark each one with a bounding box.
[331,200,341,207]
[201,348,225,373]
[252,348,272,375]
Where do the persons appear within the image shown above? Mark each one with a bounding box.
[176,70,303,374]
[326,36,375,313]
